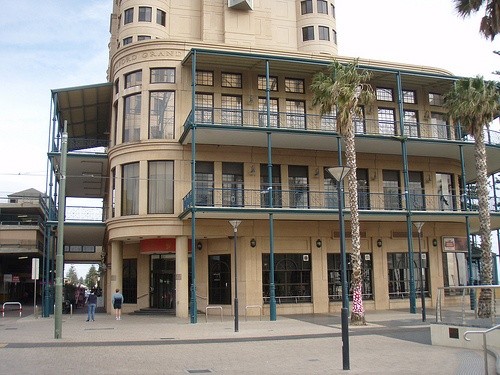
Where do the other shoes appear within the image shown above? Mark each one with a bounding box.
[92,320,95,321]
[115,317,118,320]
[86,320,89,321]
[119,318,122,320]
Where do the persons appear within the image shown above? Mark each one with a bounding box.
[112,288,123,320]
[86,290,98,321]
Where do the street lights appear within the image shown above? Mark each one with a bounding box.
[326,165,352,371]
